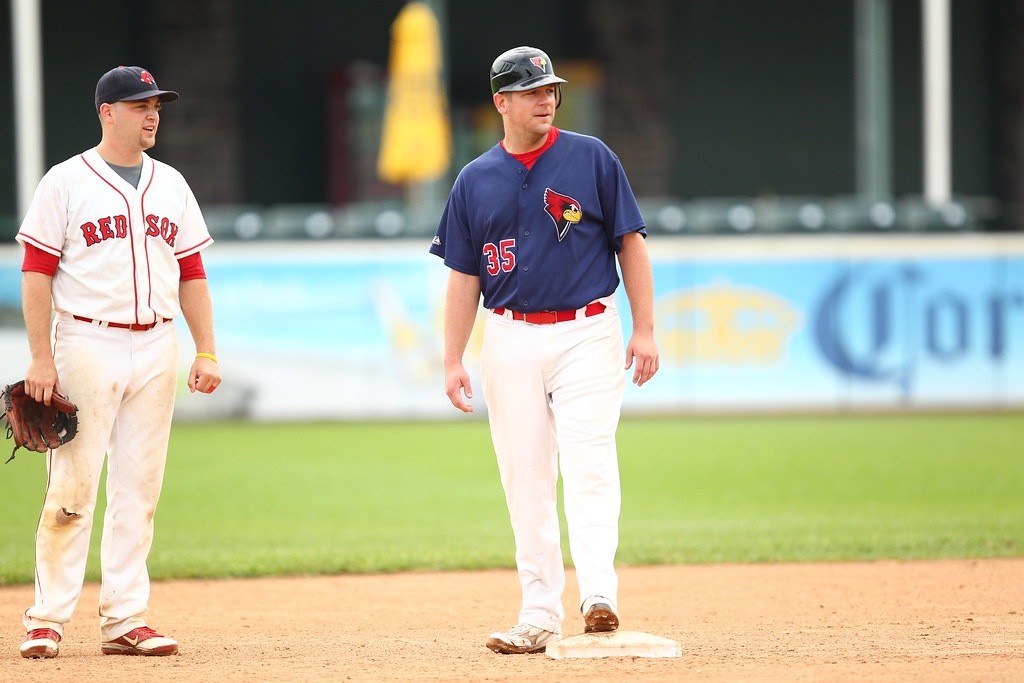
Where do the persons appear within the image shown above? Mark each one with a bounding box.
[431,47,660,654]
[15,67,222,657]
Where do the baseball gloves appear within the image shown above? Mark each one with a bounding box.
[0,379,80,462]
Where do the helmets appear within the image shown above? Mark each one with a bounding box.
[489,45,567,96]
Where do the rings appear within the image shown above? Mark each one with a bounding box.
[207,385,216,394]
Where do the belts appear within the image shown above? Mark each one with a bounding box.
[488,302,606,325]
[72,316,173,332]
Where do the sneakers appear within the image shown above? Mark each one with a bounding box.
[19,627,61,658]
[581,603,621,634]
[102,627,178,656]
[484,623,565,654]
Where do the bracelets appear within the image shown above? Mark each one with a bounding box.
[195,353,218,362]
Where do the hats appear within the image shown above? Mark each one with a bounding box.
[94,65,180,115]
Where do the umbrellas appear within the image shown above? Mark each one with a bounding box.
[377,2,453,211]
